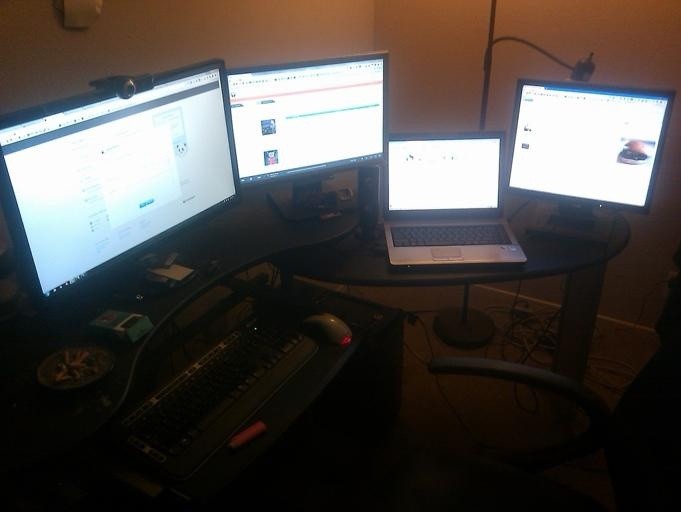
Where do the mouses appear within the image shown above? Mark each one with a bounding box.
[304,310,355,349]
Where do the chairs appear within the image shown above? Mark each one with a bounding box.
[427,240,681,510]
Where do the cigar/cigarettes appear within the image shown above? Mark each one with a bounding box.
[51,348,93,383]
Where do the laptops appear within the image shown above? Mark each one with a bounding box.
[381,131,528,267]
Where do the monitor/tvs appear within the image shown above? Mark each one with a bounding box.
[0,57,243,304]
[501,76,677,243]
[225,49,392,243]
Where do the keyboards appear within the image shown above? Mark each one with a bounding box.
[114,311,320,482]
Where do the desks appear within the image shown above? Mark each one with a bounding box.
[2,179,362,499]
[266,184,631,362]
